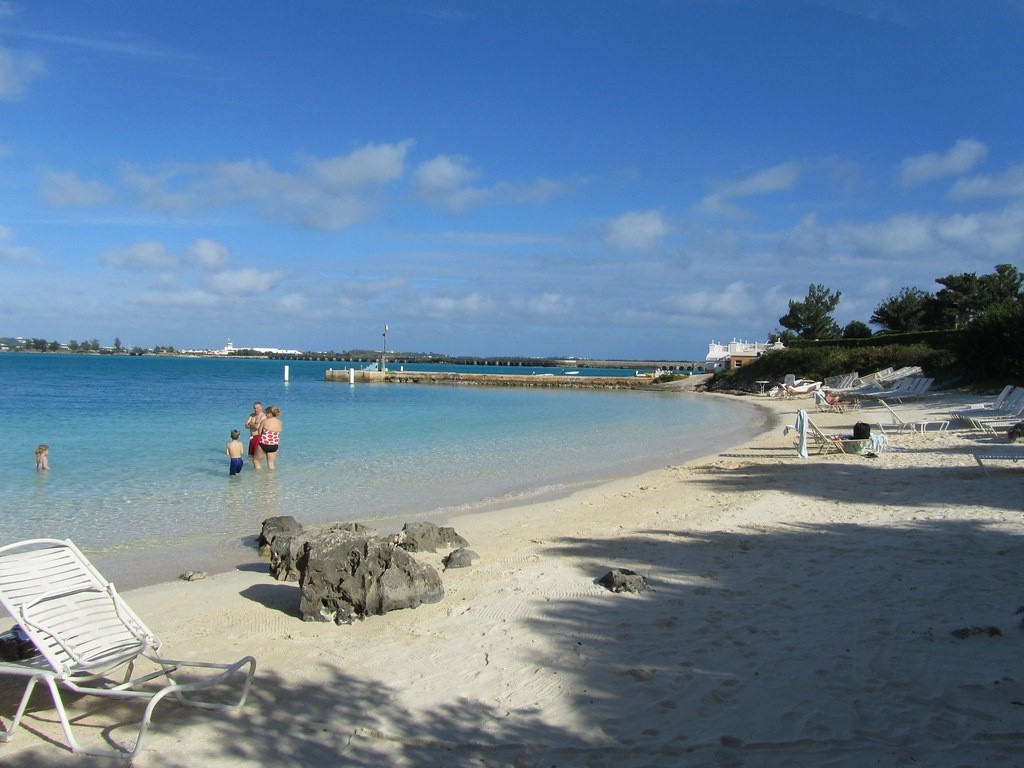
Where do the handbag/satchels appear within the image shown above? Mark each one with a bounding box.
[854,421,870,439]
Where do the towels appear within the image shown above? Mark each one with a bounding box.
[795,408,808,458]
[813,392,830,406]
[869,432,888,453]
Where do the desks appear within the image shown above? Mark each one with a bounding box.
[756,381,769,396]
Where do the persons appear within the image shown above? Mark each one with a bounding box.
[245,402,269,460]
[225,429,245,475]
[824,390,848,405]
[784,384,808,395]
[251,406,283,471]
[35,443,51,470]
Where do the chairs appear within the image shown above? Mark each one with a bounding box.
[0,538,256,759]
[783,366,1024,479]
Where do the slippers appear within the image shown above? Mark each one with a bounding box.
[862,452,878,458]
